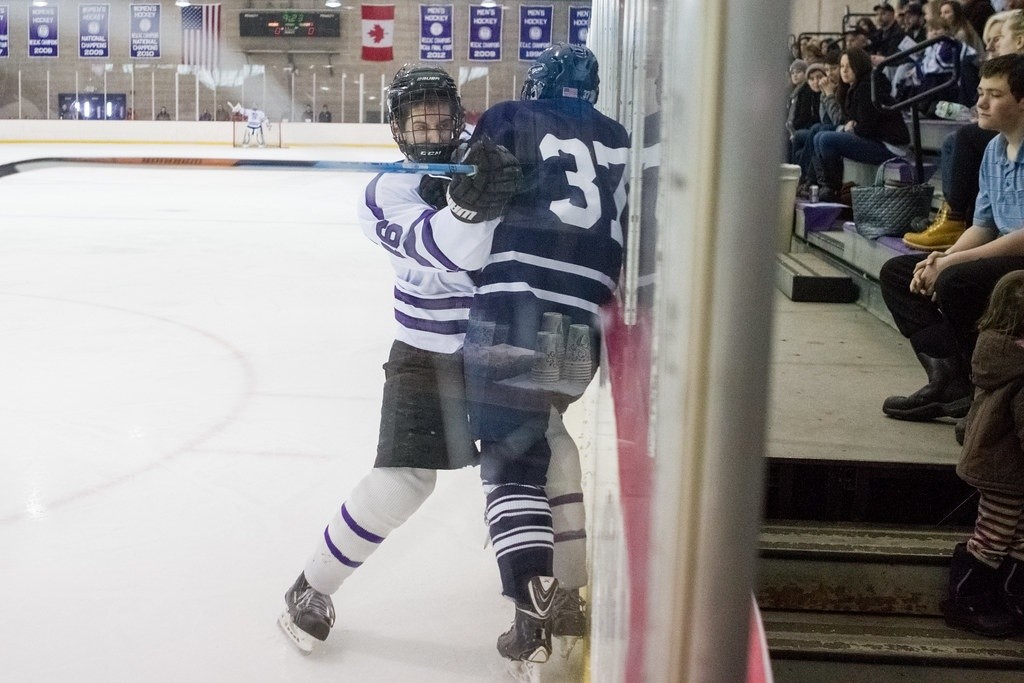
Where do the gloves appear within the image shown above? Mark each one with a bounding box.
[446,135,523,223]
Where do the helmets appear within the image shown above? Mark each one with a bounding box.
[520,42,599,106]
[386,63,467,163]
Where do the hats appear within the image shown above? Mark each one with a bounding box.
[790,59,807,74]
[874,3,893,12]
[805,63,826,80]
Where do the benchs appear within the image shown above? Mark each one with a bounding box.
[843,118,974,187]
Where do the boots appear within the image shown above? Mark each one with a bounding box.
[903,201,968,251]
[990,555,1024,624]
[882,351,972,417]
[945,542,1014,636]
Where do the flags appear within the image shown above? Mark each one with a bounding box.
[181,4,221,68]
[360,4,395,62]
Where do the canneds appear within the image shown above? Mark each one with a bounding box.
[809,185,819,203]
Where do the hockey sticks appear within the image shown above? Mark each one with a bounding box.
[0,156,480,179]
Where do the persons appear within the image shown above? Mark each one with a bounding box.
[946,270,1023,635]
[277,60,590,658]
[319,105,331,122]
[216,104,247,121]
[783,0,1024,442]
[157,106,170,120]
[199,108,212,121]
[302,104,313,122]
[448,44,632,683]
[127,108,135,120]
[240,102,271,148]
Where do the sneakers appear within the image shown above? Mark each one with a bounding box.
[551,588,587,658]
[278,572,336,654]
[496,576,558,683]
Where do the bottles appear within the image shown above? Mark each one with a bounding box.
[934,101,976,121]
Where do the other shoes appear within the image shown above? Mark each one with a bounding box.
[800,187,839,202]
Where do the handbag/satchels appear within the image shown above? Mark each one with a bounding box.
[849,156,934,239]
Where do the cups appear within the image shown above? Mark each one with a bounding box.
[530,311,597,384]
[466,316,510,377]
